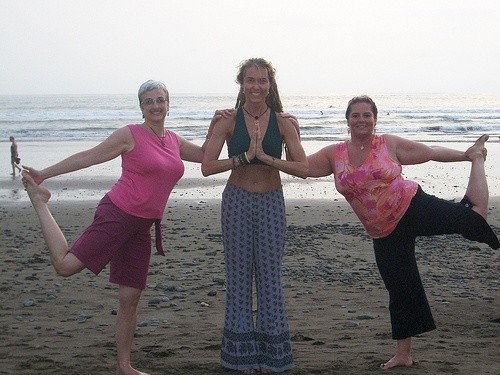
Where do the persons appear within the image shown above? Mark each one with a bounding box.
[201,57,310,375]
[21,80,235,375]
[280,95,500,368]
[10,136,21,174]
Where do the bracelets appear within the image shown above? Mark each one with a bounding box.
[238,152,251,166]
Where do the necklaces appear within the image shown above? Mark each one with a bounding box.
[243,103,268,119]
[361,144,364,151]
[144,122,165,145]
